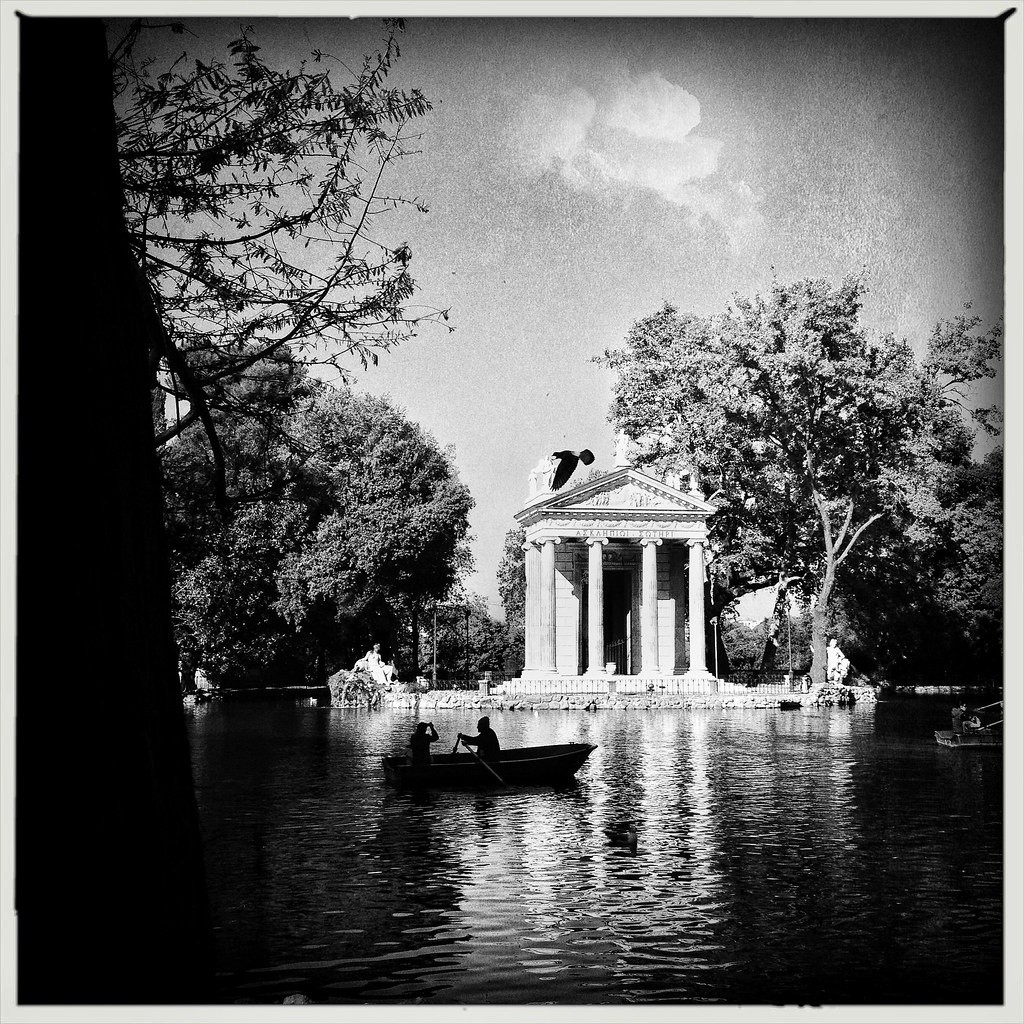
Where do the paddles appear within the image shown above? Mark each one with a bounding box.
[465,744,507,787]
[452,731,460,754]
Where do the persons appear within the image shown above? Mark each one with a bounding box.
[951,700,978,734]
[409,722,439,765]
[362,643,398,685]
[810,638,847,683]
[458,717,501,762]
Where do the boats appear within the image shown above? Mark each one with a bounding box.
[383,743,598,785]
[935,730,1003,747]
